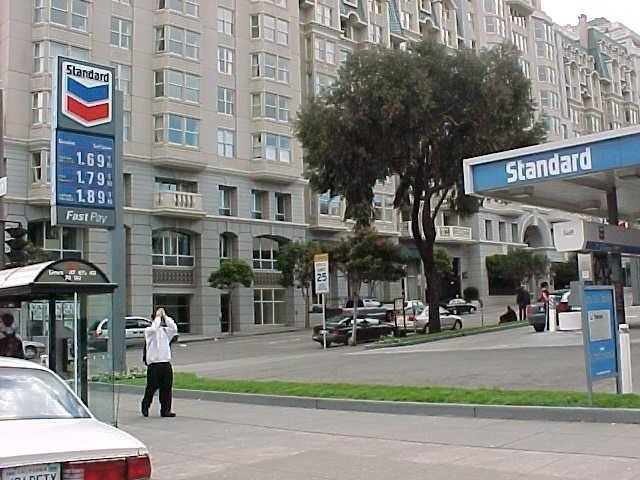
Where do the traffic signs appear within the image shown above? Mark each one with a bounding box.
[313,253,330,294]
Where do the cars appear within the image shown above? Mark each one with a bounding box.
[527,288,569,332]
[312,298,477,347]
[0,355,151,480]
[88,315,179,351]
[18,336,46,360]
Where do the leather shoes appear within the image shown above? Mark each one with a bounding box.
[142,402,148,417]
[161,412,176,417]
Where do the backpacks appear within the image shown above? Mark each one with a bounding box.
[0,337,22,358]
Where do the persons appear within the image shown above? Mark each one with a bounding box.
[540,282,549,303]
[515,285,531,321]
[1,312,25,360]
[141,307,179,419]
